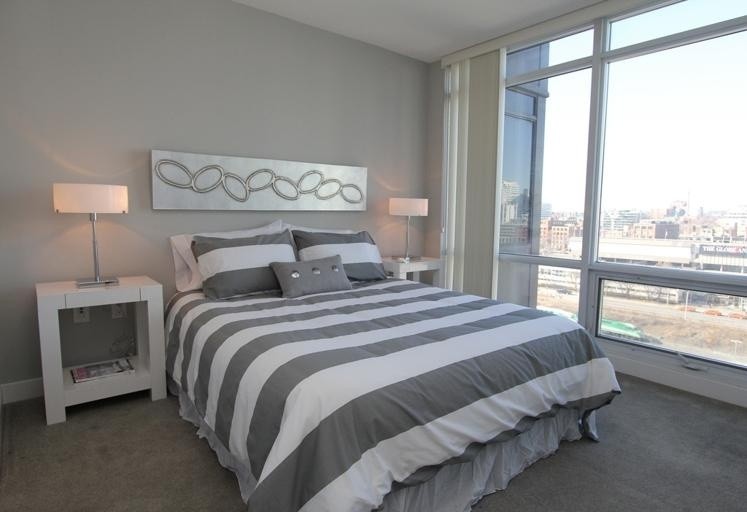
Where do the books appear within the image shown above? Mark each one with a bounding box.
[71,358,135,384]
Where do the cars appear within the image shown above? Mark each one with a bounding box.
[681,305,747,319]
[557,287,571,294]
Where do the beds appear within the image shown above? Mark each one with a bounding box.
[163,276,622,512]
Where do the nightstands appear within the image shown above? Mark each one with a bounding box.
[382,256,440,286]
[34,275,167,427]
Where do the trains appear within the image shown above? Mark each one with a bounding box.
[536,305,645,341]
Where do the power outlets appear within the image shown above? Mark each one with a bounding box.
[72,308,90,323]
[111,305,128,319]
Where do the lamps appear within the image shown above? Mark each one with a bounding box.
[52,182,129,289]
[388,197,429,261]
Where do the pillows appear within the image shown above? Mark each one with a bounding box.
[291,229,387,282]
[269,254,352,299]
[191,227,299,301]
[281,223,368,261]
[170,219,283,292]
